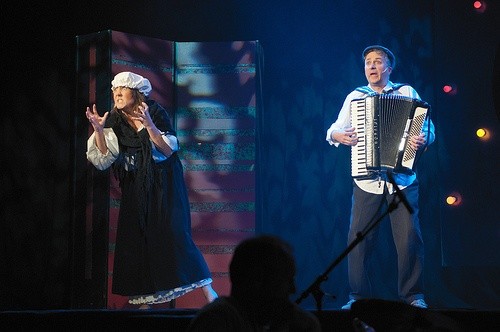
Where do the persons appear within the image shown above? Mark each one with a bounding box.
[325,45,436,311]
[85,70,219,310]
[187,234,322,332]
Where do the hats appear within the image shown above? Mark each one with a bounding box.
[362,46,395,68]
[111,71,152,97]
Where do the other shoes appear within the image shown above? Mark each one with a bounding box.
[342,298,356,309]
[411,299,428,308]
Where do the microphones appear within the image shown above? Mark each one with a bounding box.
[388,173,415,215]
[382,67,388,74]
